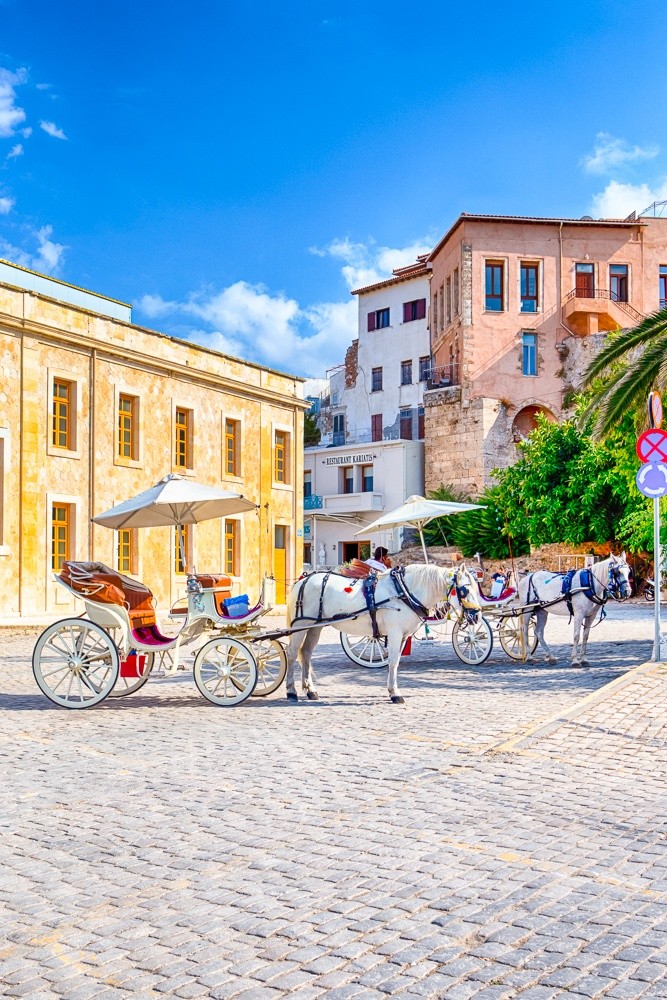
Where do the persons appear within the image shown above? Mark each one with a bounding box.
[643,559,655,593]
[363,546,392,573]
[318,543,326,566]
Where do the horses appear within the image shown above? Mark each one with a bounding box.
[518,551,632,668]
[285,563,482,703]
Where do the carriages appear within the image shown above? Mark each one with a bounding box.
[32,472,482,711]
[340,495,633,670]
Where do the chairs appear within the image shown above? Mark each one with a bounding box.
[189,572,233,592]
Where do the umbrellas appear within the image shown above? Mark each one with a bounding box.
[89,473,261,566]
[352,495,488,564]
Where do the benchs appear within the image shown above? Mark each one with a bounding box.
[468,567,484,583]
[168,595,188,618]
[61,561,156,628]
